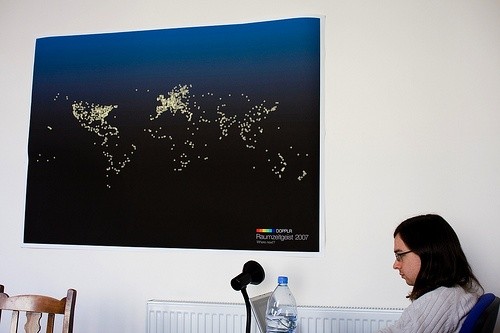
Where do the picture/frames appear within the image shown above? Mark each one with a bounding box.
[249,291,277,333]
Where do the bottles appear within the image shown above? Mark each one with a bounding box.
[265,276,298,333]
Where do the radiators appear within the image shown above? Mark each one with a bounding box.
[145,299,406,333]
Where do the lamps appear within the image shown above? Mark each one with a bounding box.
[230,260,265,333]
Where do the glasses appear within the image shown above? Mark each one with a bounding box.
[395,250,412,261]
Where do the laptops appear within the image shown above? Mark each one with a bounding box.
[248,292,276,333]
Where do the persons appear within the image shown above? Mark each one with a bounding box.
[375,213,486,333]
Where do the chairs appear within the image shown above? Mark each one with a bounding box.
[0,284,77,333]
[458,292,500,333]
